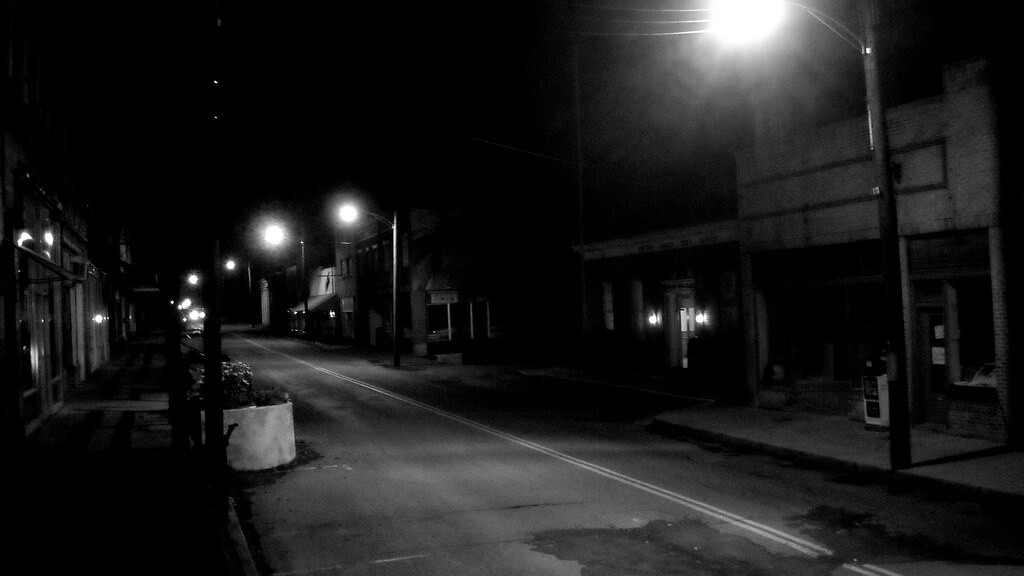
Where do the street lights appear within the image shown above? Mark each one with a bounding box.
[690,1,914,473]
[331,201,402,368]
[227,260,255,328]
[258,220,310,340]
